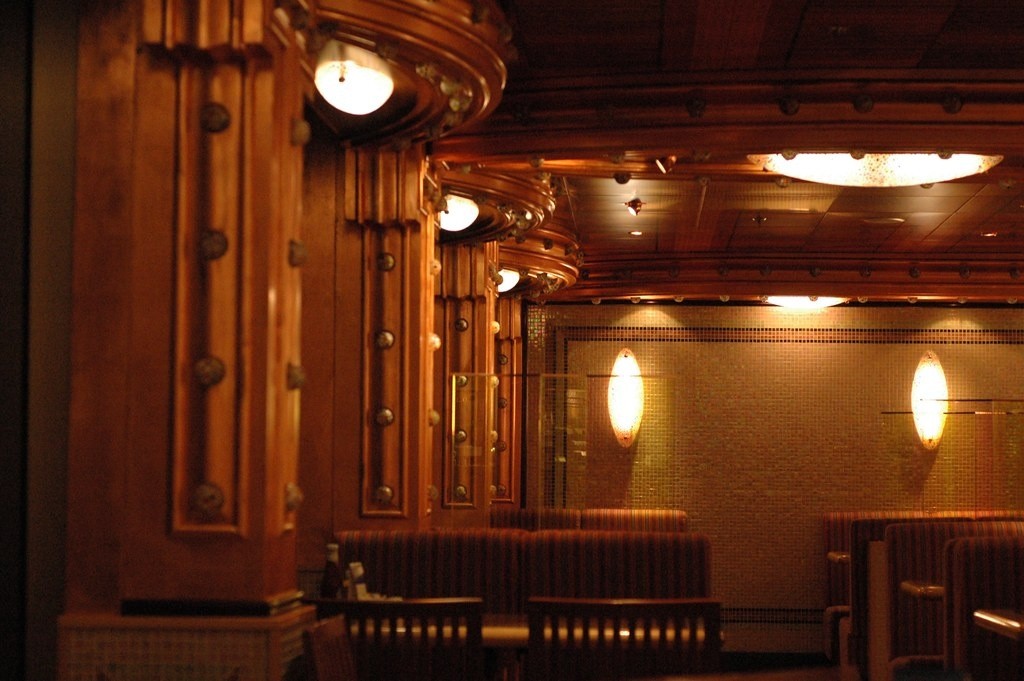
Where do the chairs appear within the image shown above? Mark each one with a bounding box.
[303,597,487,681]
[527,596,723,681]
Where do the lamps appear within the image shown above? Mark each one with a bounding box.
[607,348,644,447]
[748,152,1005,188]
[314,38,394,115]
[911,351,948,451]
[497,269,520,293]
[758,294,852,309]
[438,194,479,232]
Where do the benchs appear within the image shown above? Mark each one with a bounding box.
[822,511,1024,681]
[309,509,724,609]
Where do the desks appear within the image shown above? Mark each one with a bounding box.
[827,550,850,567]
[973,606,1024,641]
[347,618,724,663]
[900,577,946,602]
